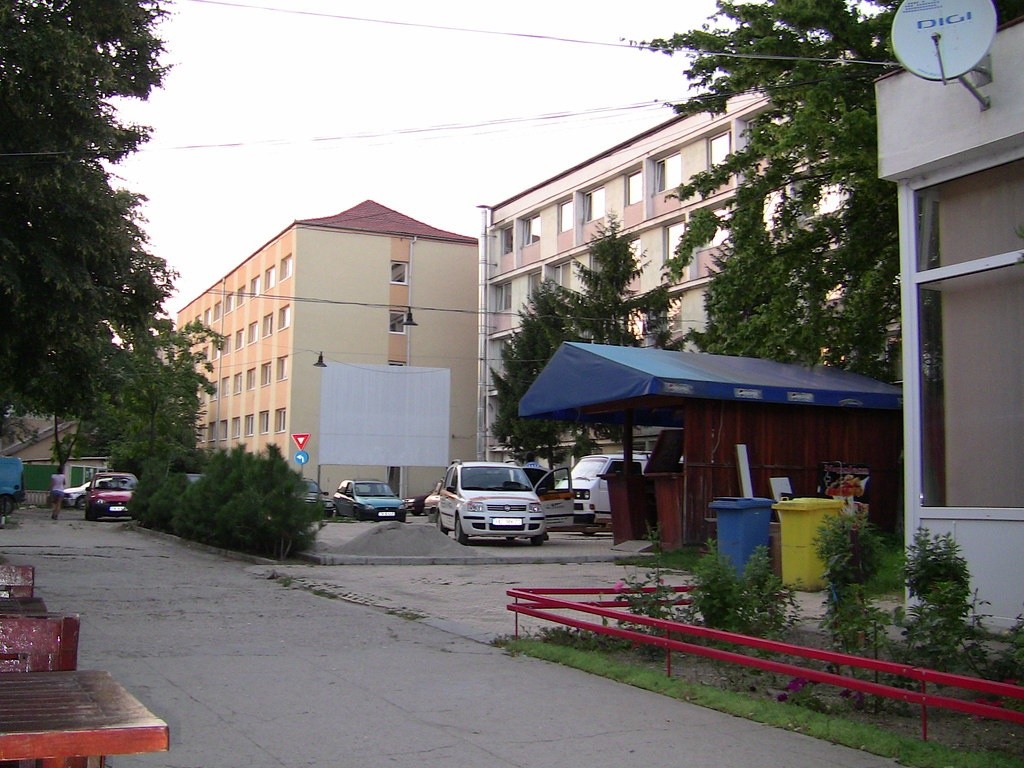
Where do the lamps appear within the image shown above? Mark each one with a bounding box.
[402,306,418,326]
[313,351,327,367]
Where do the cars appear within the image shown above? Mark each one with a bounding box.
[86,473,139,521]
[303,478,336,518]
[402,482,443,516]
[333,479,406,522]
[0,457,26,516]
[63,478,113,510]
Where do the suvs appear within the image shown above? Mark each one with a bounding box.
[435,459,575,546]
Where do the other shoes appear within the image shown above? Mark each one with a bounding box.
[52,514,58,521]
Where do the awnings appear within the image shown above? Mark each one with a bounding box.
[518,341,903,419]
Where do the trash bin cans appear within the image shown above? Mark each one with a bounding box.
[771,496,844,592]
[708,496,773,578]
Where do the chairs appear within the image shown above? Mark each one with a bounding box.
[0,565,35,597]
[99,481,109,488]
[0,613,81,673]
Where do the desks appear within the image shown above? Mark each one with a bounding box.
[0,672,170,768]
[0,596,49,619]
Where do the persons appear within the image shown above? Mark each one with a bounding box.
[48,467,65,520]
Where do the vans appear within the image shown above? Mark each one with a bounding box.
[555,451,684,536]
